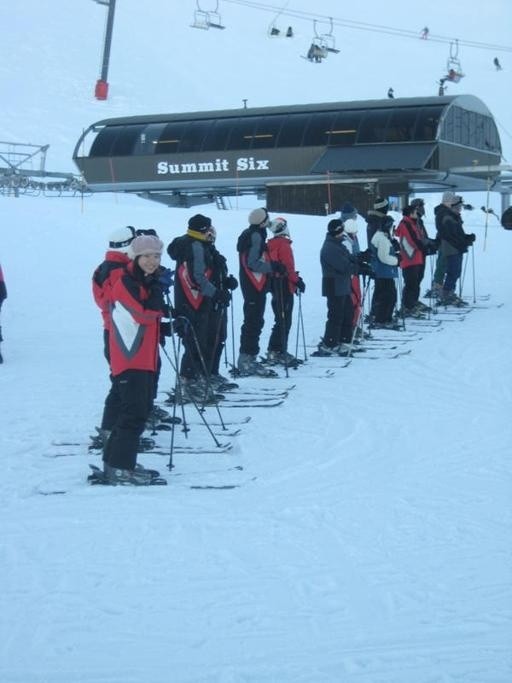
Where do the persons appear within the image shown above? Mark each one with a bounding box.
[145,267,188,427]
[167,214,238,401]
[388,87,395,99]
[318,46,329,58]
[418,25,431,40]
[236,207,305,374]
[320,191,477,353]
[92,226,135,447]
[310,45,322,62]
[494,57,502,71]
[100,234,184,483]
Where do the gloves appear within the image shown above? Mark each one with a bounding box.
[423,238,439,254]
[296,276,305,292]
[214,275,237,303]
[271,263,285,272]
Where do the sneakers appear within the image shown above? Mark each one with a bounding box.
[238,351,294,374]
[432,281,461,304]
[176,373,227,402]
[103,461,153,484]
[318,341,348,354]
[145,405,171,428]
[370,301,427,327]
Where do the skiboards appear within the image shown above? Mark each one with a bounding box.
[216,291,505,379]
[34,376,296,489]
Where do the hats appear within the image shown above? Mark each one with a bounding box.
[109,226,162,257]
[329,202,357,235]
[188,214,211,241]
[249,208,290,237]
[442,191,462,209]
[380,216,394,231]
[374,198,389,214]
